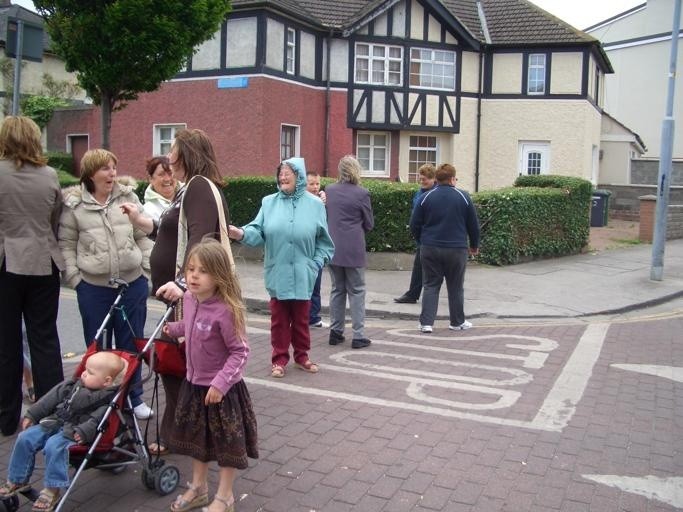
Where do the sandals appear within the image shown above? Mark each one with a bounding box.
[202,494,234,512]
[272,365,284,378]
[171,481,209,512]
[0,481,31,499]
[32,489,62,512]
[296,360,318,372]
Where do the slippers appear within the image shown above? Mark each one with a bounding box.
[148,439,170,455]
[26,387,35,402]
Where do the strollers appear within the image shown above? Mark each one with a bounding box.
[0,279,183,512]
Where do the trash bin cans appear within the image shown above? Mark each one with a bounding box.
[591,189,611,227]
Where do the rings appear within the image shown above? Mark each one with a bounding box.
[169,295,175,299]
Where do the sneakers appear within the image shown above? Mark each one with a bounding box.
[310,313,371,348]
[420,324,432,332]
[449,321,472,330]
[134,402,154,420]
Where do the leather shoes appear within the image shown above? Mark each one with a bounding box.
[394,294,416,303]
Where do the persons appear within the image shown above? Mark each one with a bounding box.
[57,149,153,418]
[0,351,123,511]
[163,243,257,512]
[119,130,231,455]
[21,312,37,403]
[139,156,191,282]
[306,171,325,328]
[227,157,336,377]
[392,165,437,305]
[0,115,60,435]
[409,165,477,332]
[326,154,374,348]
